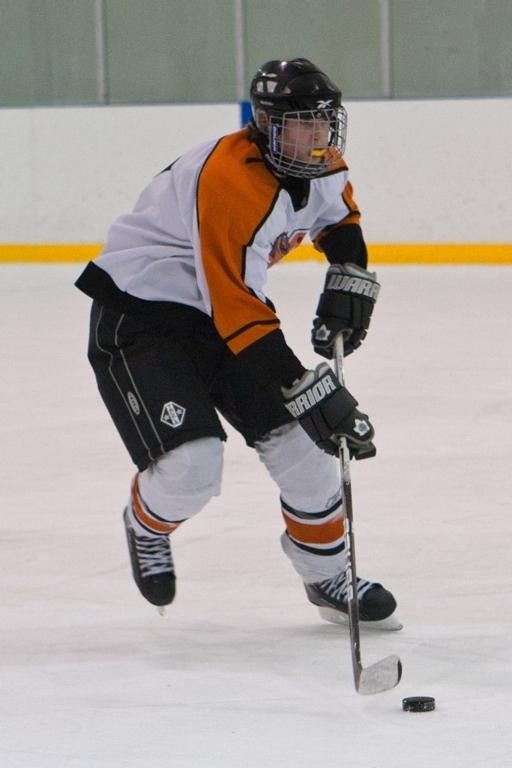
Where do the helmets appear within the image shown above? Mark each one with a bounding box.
[249,57,348,178]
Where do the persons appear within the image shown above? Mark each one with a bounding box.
[75,58,397,622]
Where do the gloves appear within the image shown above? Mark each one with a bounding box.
[279,362,377,461]
[313,261,381,358]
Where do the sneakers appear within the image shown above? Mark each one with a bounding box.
[122,507,174,605]
[303,571,396,622]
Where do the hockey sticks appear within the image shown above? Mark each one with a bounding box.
[334,334,403,694]
